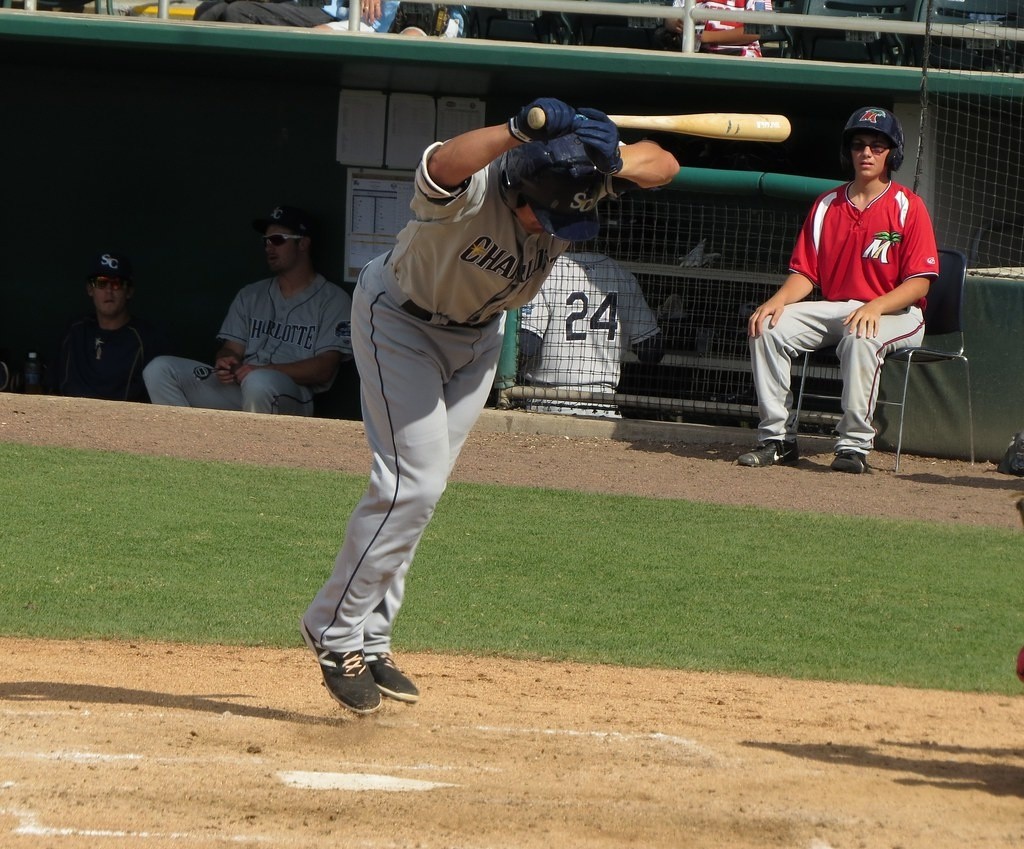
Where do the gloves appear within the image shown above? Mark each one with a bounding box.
[510,97,623,176]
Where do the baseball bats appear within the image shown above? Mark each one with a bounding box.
[526,104,792,144]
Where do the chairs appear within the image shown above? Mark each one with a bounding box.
[473,0,1024,70]
[792,246,975,472]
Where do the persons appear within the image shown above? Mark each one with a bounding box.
[298,98,680,716]
[515,231,677,418]
[35,247,174,405]
[13,0,774,35]
[738,107,939,475]
[141,203,353,416]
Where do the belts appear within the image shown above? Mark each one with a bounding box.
[402,299,490,329]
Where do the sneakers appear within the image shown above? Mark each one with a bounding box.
[301,621,420,716]
[831,449,867,474]
[738,438,799,467]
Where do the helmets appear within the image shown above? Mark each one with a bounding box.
[841,107,903,169]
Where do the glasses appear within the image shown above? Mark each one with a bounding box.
[262,233,302,246]
[850,142,891,155]
[90,279,130,291]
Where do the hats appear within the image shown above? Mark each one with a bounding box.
[89,251,134,278]
[251,205,327,234]
[508,133,604,242]
[732,230,768,260]
